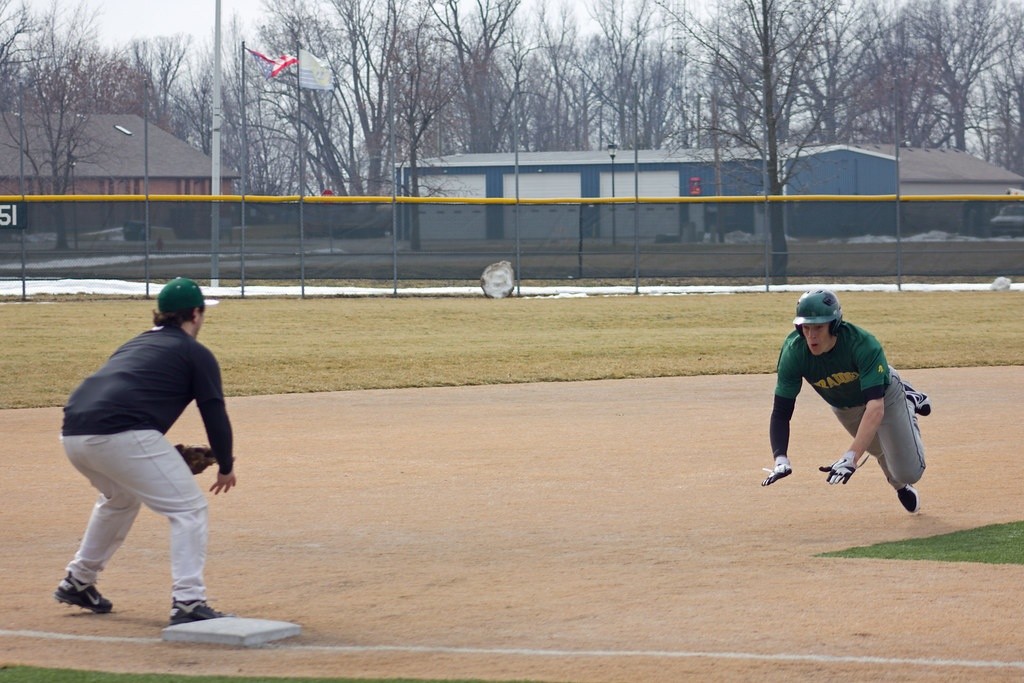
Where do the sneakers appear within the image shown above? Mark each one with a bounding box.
[52,572,113,612]
[896,484,920,513]
[903,381,931,416]
[170,597,236,625]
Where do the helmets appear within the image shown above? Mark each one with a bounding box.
[793,289,843,338]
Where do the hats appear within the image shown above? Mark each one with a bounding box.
[157,277,220,309]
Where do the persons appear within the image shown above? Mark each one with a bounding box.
[56,276,237,626]
[755,288,932,516]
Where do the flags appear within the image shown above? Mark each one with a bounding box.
[298,49,336,93]
[244,47,299,79]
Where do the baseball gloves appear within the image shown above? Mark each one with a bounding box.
[175,443,236,475]
